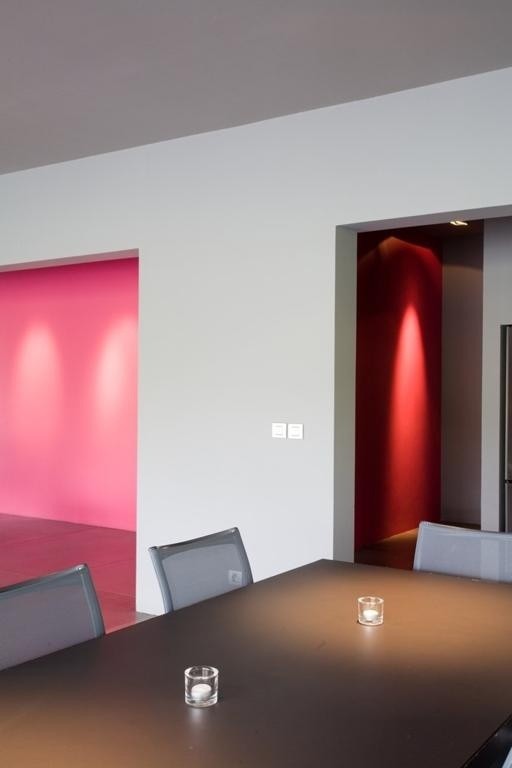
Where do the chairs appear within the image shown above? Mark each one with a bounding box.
[0,527,254,667]
[411,520,511,586]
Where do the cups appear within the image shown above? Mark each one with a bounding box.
[184,665,219,708]
[357,596,384,626]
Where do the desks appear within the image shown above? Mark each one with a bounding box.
[0,558,512,767]
